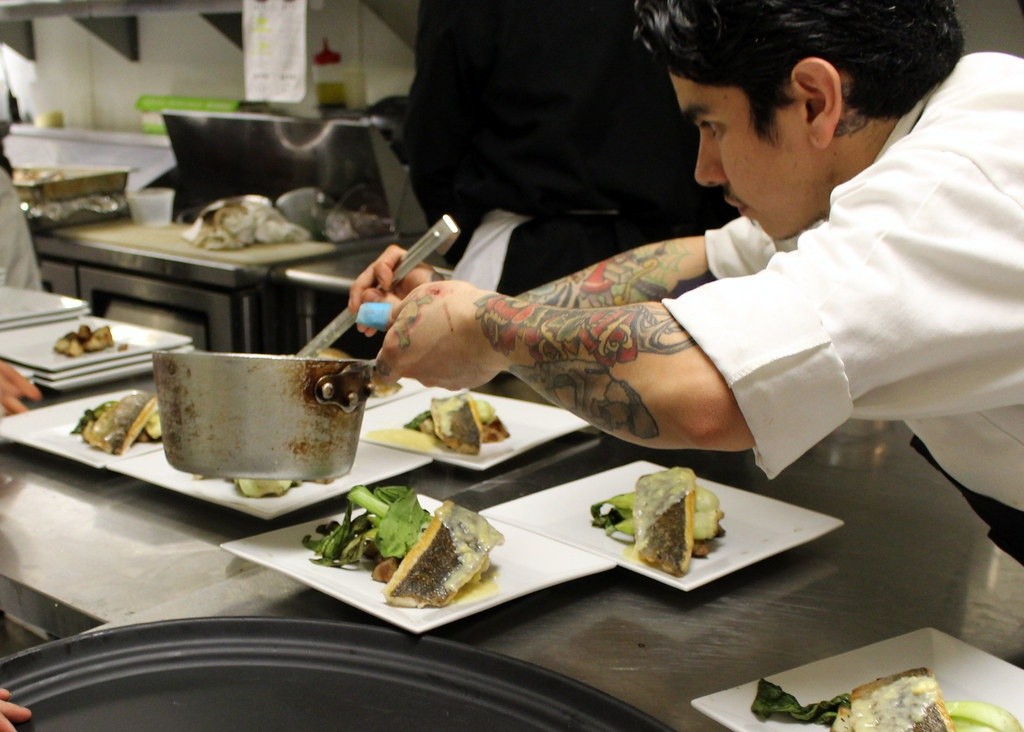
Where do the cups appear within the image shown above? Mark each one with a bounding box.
[128,187,175,228]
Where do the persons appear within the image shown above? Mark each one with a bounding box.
[349,0,1024,569]
[0,167,44,732]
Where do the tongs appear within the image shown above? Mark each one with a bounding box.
[296,213,461,357]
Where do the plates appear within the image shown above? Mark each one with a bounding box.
[359,387,592,472]
[691,625,1024,732]
[220,492,619,634]
[106,438,433,520]
[0,286,196,420]
[0,389,162,470]
[477,459,846,593]
[364,377,427,409]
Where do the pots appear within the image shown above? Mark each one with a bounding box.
[150,347,372,484]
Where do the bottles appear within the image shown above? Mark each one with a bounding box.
[307,32,343,118]
